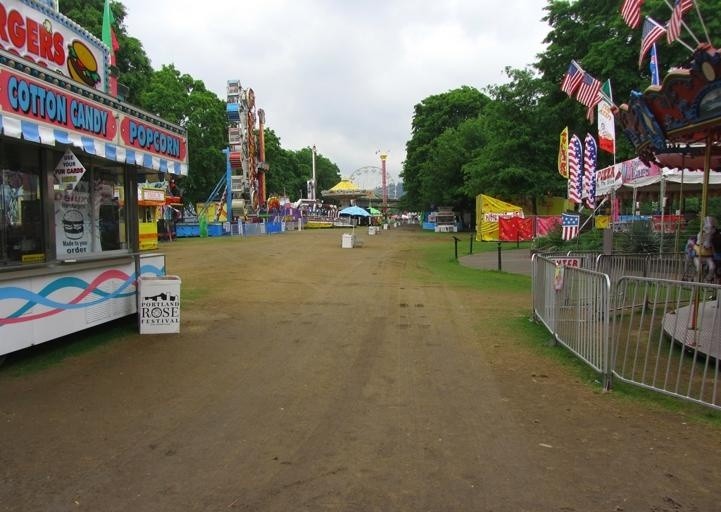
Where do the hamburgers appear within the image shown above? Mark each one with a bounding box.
[67,40,100,86]
[62,210,84,240]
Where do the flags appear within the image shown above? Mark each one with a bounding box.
[561,61,584,100]
[560,212,580,241]
[638,13,668,71]
[595,80,616,152]
[620,0,644,30]
[666,0,693,44]
[647,43,663,87]
[109,26,121,51]
[99,0,117,56]
[585,96,603,127]
[574,71,600,107]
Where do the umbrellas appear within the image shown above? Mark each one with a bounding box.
[365,206,383,216]
[340,205,372,232]
